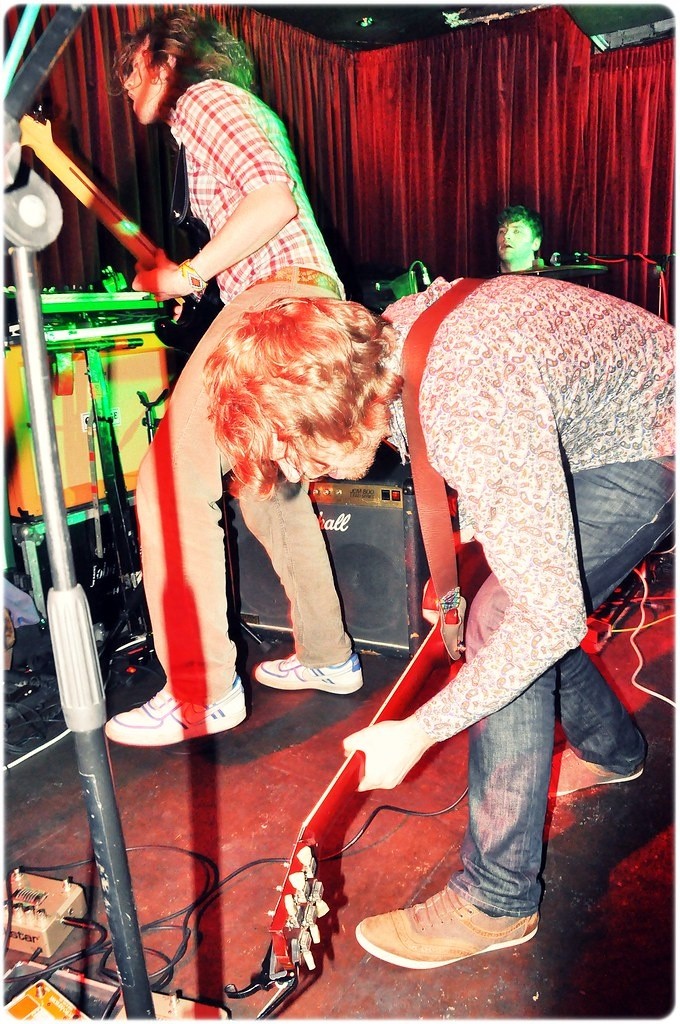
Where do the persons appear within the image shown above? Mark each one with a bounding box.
[205,273,676,969]
[103,12,364,746]
[496,204,541,273]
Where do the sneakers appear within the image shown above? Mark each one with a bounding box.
[355,883,540,969]
[254,654,364,694]
[547,748,643,797]
[105,674,246,747]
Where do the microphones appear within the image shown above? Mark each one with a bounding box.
[549,251,588,266]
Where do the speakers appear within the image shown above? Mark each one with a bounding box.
[225,457,435,660]
[5,331,174,520]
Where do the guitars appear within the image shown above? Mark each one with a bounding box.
[17,108,225,358]
[269,483,493,978]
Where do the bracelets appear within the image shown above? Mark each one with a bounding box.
[177,259,208,302]
